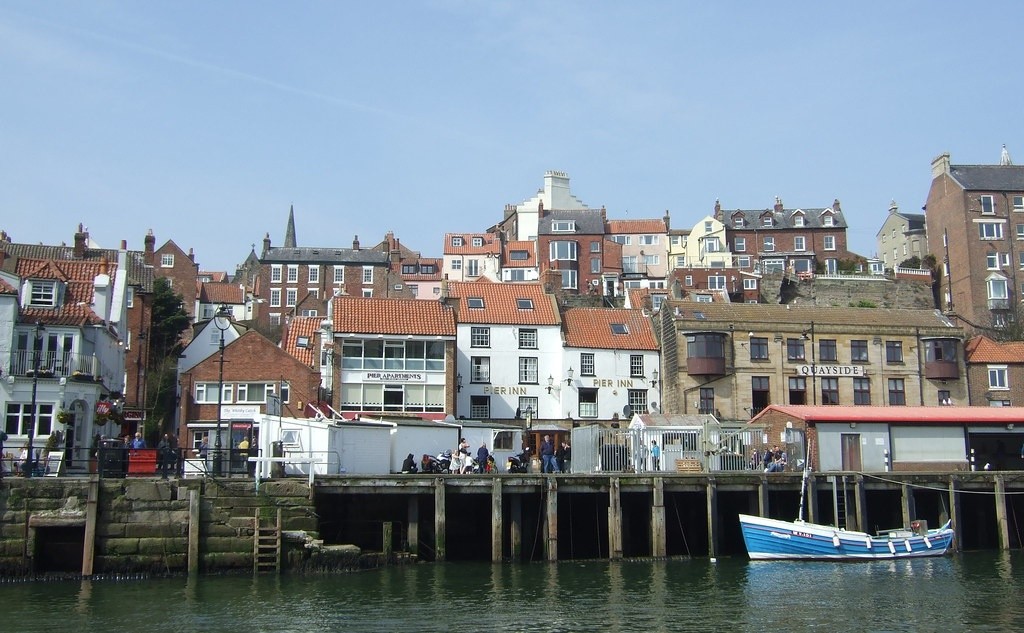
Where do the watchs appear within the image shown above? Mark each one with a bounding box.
[554,451,557,452]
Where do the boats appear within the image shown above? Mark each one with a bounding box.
[737,513,955,556]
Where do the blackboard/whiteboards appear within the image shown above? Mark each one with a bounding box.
[43,451,67,476]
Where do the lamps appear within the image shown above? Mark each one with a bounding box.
[649,369,659,387]
[565,366,575,386]
[545,375,554,393]
[456,372,463,393]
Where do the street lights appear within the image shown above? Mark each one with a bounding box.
[25,319,46,478]
[798,319,817,406]
[213,301,232,478]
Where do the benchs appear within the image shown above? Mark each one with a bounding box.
[676,460,701,473]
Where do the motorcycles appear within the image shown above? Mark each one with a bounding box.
[472,451,500,474]
[421,449,452,474]
[506,447,531,473]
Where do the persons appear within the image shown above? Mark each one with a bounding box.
[123,434,132,447]
[248,435,258,457]
[130,431,145,449]
[97,435,109,473]
[237,436,249,461]
[198,436,208,463]
[748,445,787,472]
[449,438,477,474]
[558,441,571,473]
[401,453,418,474]
[540,434,561,473]
[650,440,660,471]
[156,433,178,468]
[421,454,438,474]
[475,442,489,474]
[635,438,648,471]
[0,430,37,479]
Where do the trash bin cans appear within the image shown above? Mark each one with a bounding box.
[96,438,129,479]
[272,440,287,477]
